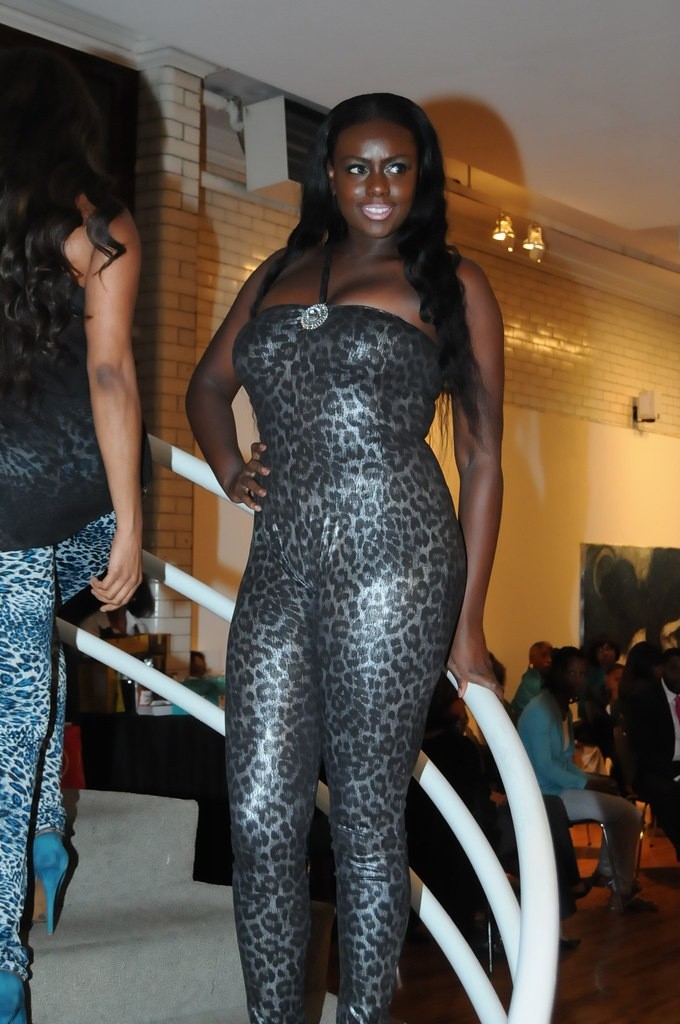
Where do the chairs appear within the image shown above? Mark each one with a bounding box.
[569,818,627,917]
[611,719,655,884]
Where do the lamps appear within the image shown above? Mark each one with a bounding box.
[522,224,545,251]
[492,214,516,240]
[633,389,661,423]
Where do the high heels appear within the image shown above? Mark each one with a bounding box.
[0,970,27,1023]
[21,833,70,935]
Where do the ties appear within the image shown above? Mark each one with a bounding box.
[674,696,680,722]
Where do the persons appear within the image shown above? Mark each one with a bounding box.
[103,609,680,958]
[0,48,150,1024]
[184,91,505,1024]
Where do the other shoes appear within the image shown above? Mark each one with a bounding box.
[592,871,640,888]
[608,897,658,912]
[560,936,580,947]
